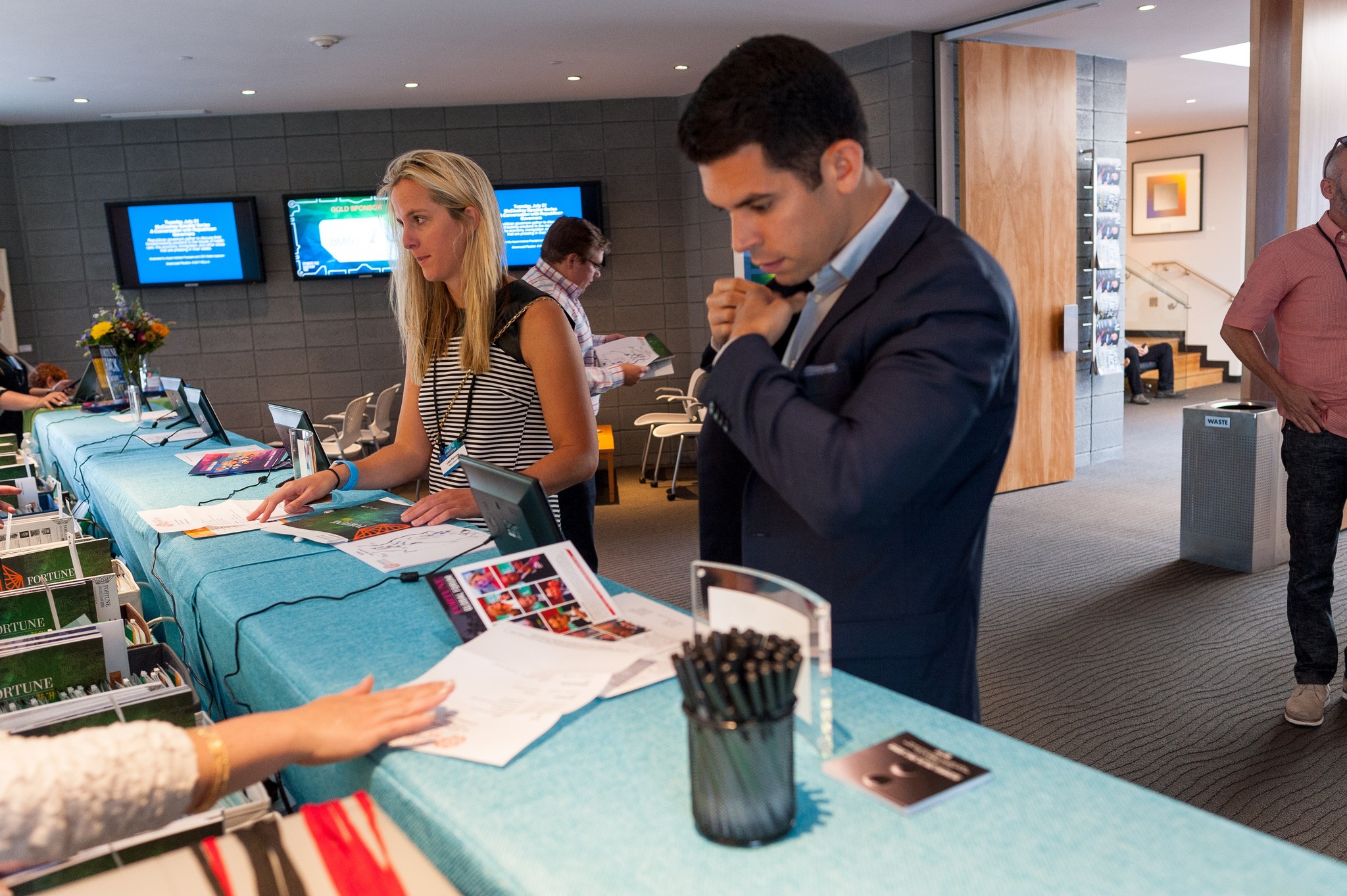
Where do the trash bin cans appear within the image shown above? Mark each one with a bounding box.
[1179,397,1291,574]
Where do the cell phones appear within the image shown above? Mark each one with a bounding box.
[62,376,81,391]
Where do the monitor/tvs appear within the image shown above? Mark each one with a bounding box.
[104,196,267,290]
[457,453,564,556]
[489,181,605,271]
[280,191,410,282]
[268,402,331,474]
[160,376,197,424]
[184,386,231,445]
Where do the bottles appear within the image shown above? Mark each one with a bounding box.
[20,432,45,478]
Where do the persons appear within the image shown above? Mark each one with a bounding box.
[0,486,21,529]
[465,552,595,633]
[1124,339,1188,404]
[0,289,77,448]
[0,674,457,875]
[676,36,1021,725]
[1220,136,1347,726]
[247,150,599,535]
[520,214,650,573]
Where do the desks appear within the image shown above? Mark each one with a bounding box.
[31,404,1347,896]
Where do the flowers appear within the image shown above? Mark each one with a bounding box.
[71,279,177,383]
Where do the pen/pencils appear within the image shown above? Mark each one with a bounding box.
[671,627,804,843]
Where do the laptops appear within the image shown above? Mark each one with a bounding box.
[52,358,97,404]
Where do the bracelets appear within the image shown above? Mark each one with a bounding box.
[326,468,340,489]
[329,460,359,490]
[185,724,230,815]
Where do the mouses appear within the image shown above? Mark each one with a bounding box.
[50,399,74,407]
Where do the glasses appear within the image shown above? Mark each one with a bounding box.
[577,254,602,273]
[1324,136,1347,179]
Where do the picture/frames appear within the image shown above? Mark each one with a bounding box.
[1130,153,1205,236]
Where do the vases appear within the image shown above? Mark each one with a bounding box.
[128,384,143,425]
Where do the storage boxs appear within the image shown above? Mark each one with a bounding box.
[0,426,470,896]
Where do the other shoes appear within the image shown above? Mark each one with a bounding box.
[1156,390,1187,399]
[1131,394,1150,404]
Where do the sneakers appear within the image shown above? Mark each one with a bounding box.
[1285,684,1330,725]
[1341,678,1347,700]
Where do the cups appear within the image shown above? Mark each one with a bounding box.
[680,691,797,848]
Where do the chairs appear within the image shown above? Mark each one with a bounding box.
[631,365,707,484]
[1124,360,1161,393]
[649,396,707,502]
